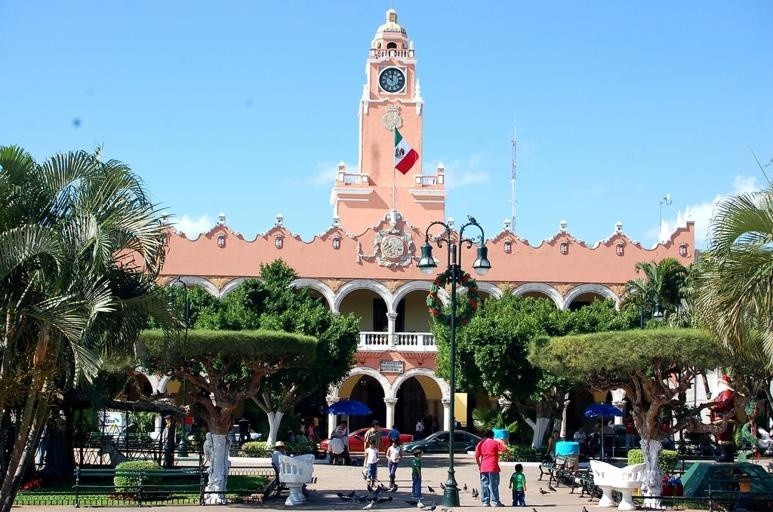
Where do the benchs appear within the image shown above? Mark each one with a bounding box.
[538,452,602,502]
[272,462,310,499]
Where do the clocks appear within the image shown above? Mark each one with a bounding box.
[379,65,407,94]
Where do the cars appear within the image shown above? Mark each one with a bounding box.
[396,429,487,457]
[230,422,265,442]
[317,425,415,455]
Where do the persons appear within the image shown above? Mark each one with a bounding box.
[709,372,735,461]
[385,436,404,488]
[509,463,528,506]
[360,439,380,484]
[408,444,427,497]
[475,430,508,507]
[153,413,685,471]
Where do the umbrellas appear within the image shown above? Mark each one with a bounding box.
[326,398,371,446]
[583,401,623,457]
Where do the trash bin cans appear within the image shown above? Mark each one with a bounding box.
[491,429,509,444]
[555,442,580,470]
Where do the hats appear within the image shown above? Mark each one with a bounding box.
[269,441,285,449]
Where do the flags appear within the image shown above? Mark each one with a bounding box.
[395,127,418,175]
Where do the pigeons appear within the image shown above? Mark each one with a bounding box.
[362,472,367,480]
[539,482,557,495]
[456,488,462,492]
[533,508,537,512]
[464,484,468,491]
[440,483,445,489]
[336,482,399,509]
[403,498,452,512]
[581,506,588,512]
[428,486,436,493]
[471,488,479,498]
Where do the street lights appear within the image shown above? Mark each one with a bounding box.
[415,214,496,507]
[165,277,194,458]
